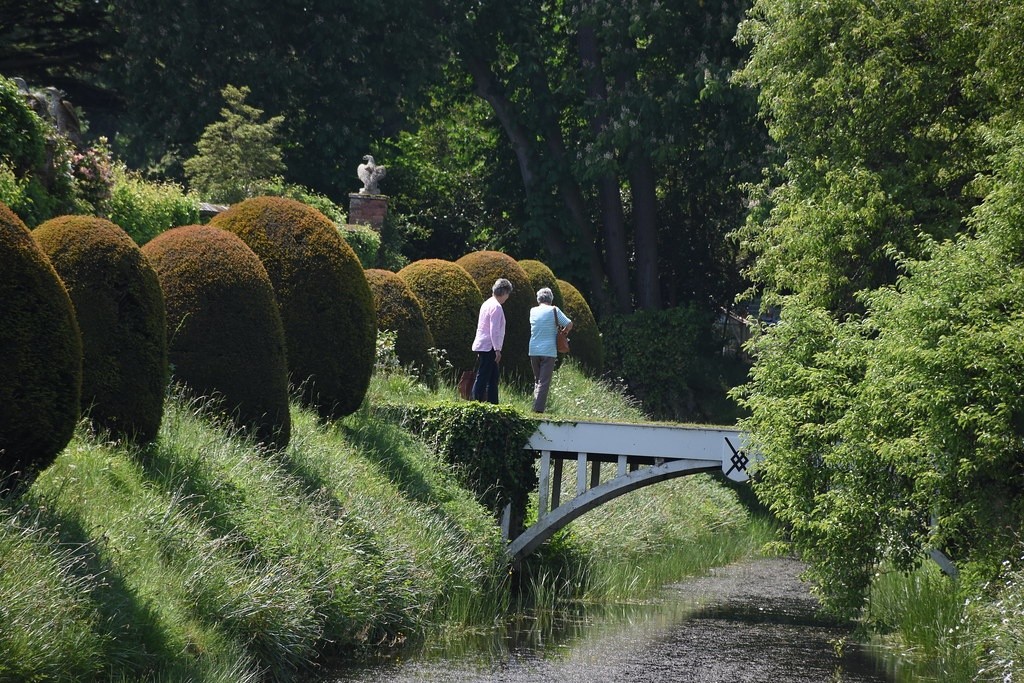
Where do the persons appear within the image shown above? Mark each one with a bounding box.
[529,287,572,413]
[471,278,512,403]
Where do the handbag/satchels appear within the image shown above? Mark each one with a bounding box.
[553,305,570,354]
[459,357,480,401]
